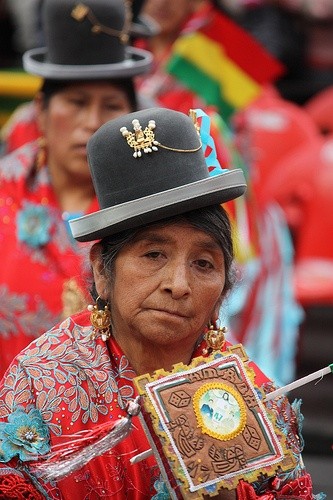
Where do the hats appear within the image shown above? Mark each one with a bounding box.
[23,0,154,78]
[67,108,246,242]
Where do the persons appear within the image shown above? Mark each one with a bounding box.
[0,0,332,370]
[0,198,303,500]
